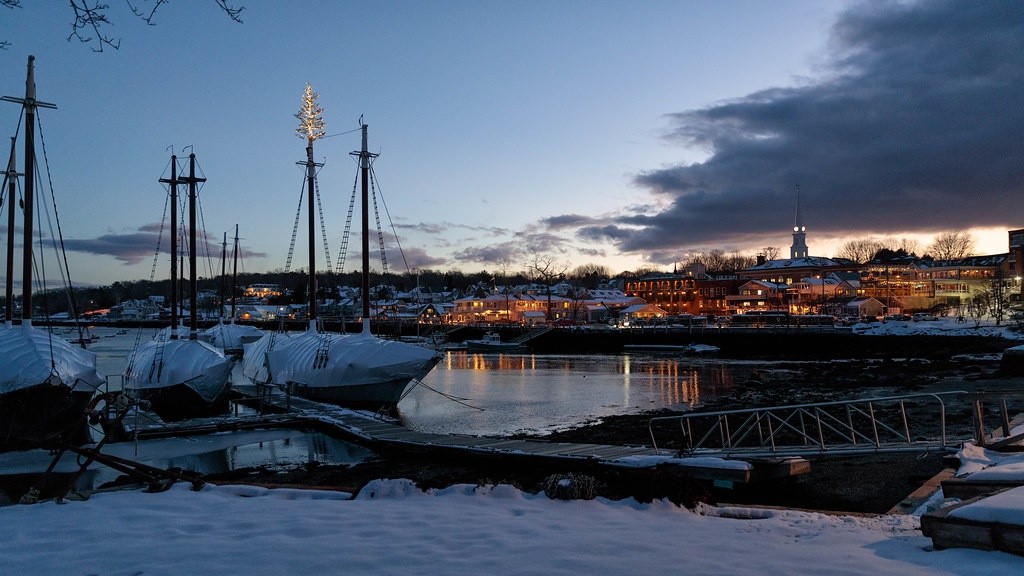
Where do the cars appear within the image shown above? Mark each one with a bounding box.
[599,314,692,326]
[886,311,911,321]
[554,317,586,326]
[840,313,879,327]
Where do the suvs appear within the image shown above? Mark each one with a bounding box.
[913,313,938,322]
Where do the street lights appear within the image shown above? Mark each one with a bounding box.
[817,275,824,307]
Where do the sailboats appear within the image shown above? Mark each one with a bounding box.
[0,55,447,438]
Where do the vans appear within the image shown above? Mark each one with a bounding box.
[718,316,732,323]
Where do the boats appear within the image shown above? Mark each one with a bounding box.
[465,328,519,351]
[680,341,721,358]
[423,329,467,351]
